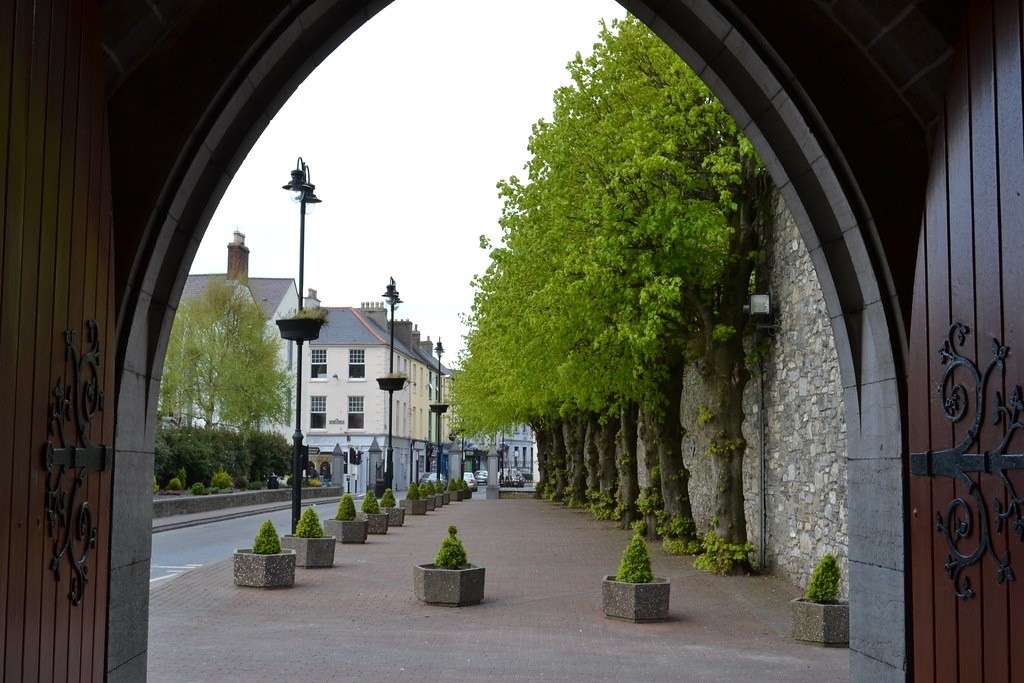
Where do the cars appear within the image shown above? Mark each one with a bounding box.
[419,472,448,487]
[464,472,478,492]
[473,469,489,485]
[498,467,526,487]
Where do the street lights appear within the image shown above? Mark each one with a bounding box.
[382,275,404,490]
[282,156,323,538]
[434,335,446,483]
